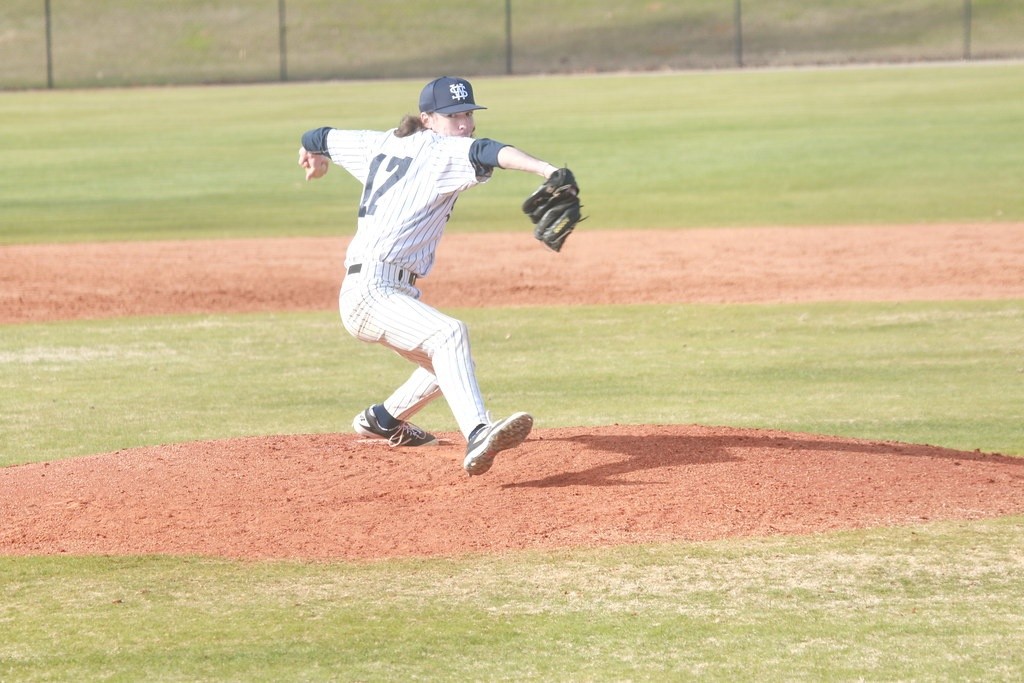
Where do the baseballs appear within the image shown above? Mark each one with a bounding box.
[306,159,329,179]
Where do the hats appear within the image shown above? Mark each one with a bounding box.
[419,76,488,116]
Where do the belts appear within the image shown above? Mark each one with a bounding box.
[348,264,417,286]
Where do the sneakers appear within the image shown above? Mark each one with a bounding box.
[352,404,437,448]
[464,410,534,477]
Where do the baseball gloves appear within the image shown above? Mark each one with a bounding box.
[520,168,581,254]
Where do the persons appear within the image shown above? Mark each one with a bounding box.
[297,75,581,475]
